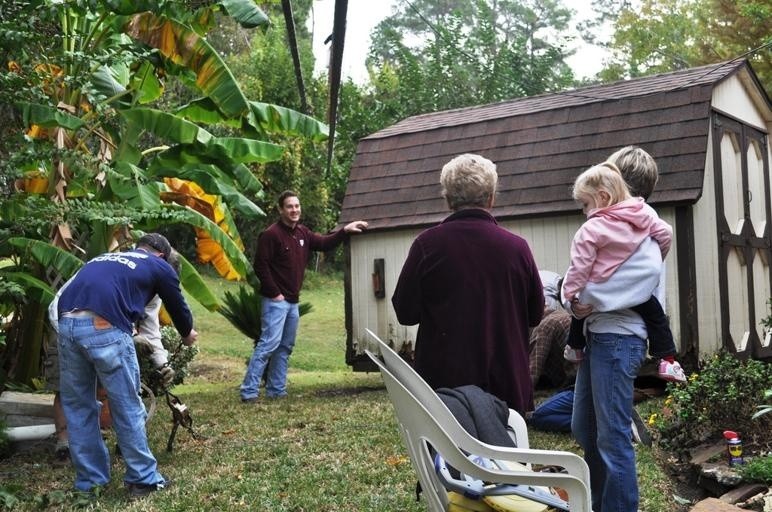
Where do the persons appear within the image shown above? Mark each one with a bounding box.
[47,250,183,463]
[55,231,199,498]
[388,151,547,420]
[527,306,656,450]
[567,141,664,512]
[532,265,564,312]
[562,161,690,385]
[237,189,369,404]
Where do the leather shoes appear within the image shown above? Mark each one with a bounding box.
[128,479,171,496]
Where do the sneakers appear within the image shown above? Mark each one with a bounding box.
[628,408,652,447]
[53,444,72,465]
[150,366,174,397]
[657,359,687,383]
[563,345,584,362]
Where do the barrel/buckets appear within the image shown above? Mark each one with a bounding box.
[52,388,114,433]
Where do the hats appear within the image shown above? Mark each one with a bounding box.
[138,233,172,258]
